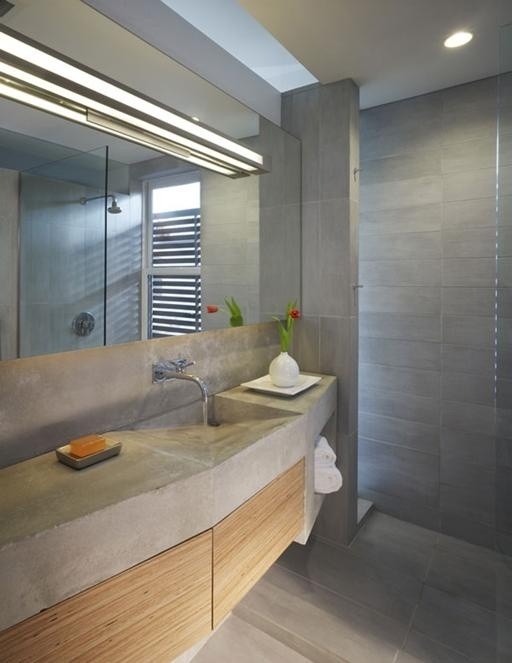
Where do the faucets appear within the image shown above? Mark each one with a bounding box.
[153,357,208,404]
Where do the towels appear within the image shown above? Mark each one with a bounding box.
[315,436,337,469]
[314,466,344,495]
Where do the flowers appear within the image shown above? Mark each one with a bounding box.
[270,299,300,351]
[207,297,244,327]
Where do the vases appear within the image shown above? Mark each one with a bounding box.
[270,350,300,388]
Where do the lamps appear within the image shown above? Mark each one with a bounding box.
[1,79,249,179]
[0,23,273,175]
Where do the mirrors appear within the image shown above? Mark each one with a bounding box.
[0,0,302,363]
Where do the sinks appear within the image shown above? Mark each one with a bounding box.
[122,389,304,436]
